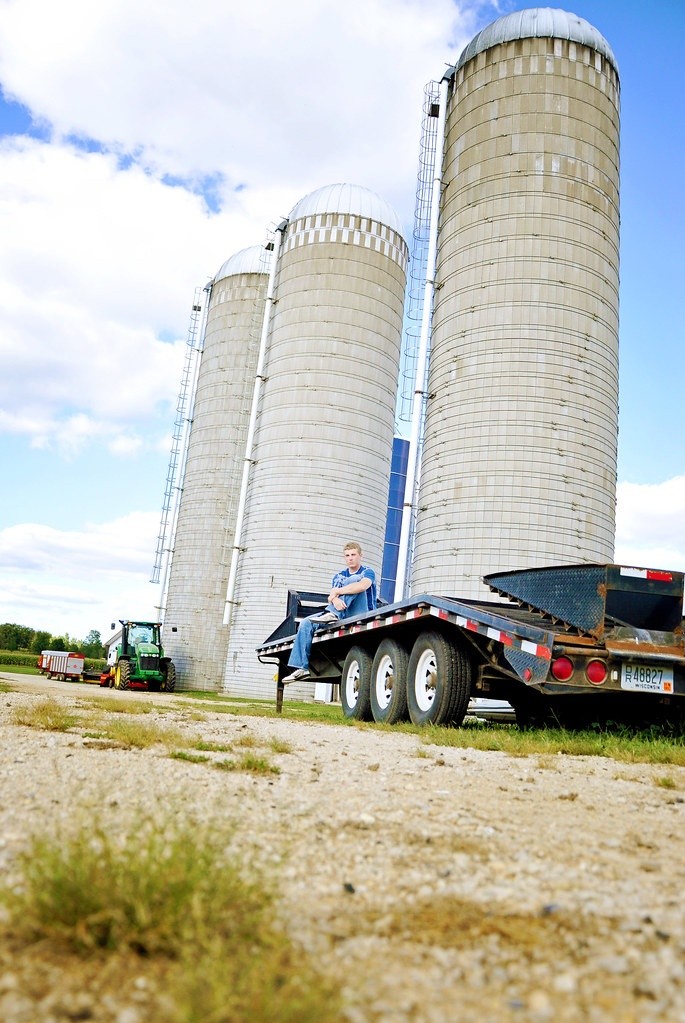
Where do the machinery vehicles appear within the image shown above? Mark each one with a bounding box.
[110,620,176,692]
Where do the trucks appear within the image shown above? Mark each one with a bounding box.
[46,650,85,683]
[36,649,59,674]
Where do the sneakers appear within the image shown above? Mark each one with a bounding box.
[282,669,310,684]
[309,613,337,623]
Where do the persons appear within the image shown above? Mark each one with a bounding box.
[86,661,95,673]
[282,542,378,684]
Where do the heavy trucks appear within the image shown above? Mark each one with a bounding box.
[258,562,685,741]
